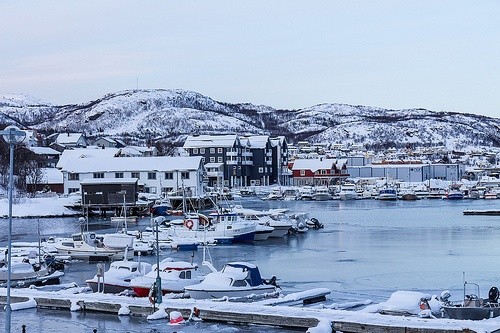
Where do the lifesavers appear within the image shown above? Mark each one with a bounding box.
[185,219,194,229]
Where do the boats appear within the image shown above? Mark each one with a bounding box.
[449,190,464,198]
[86,221,282,299]
[0,170,324,287]
[260,181,418,201]
[381,272,500,320]
